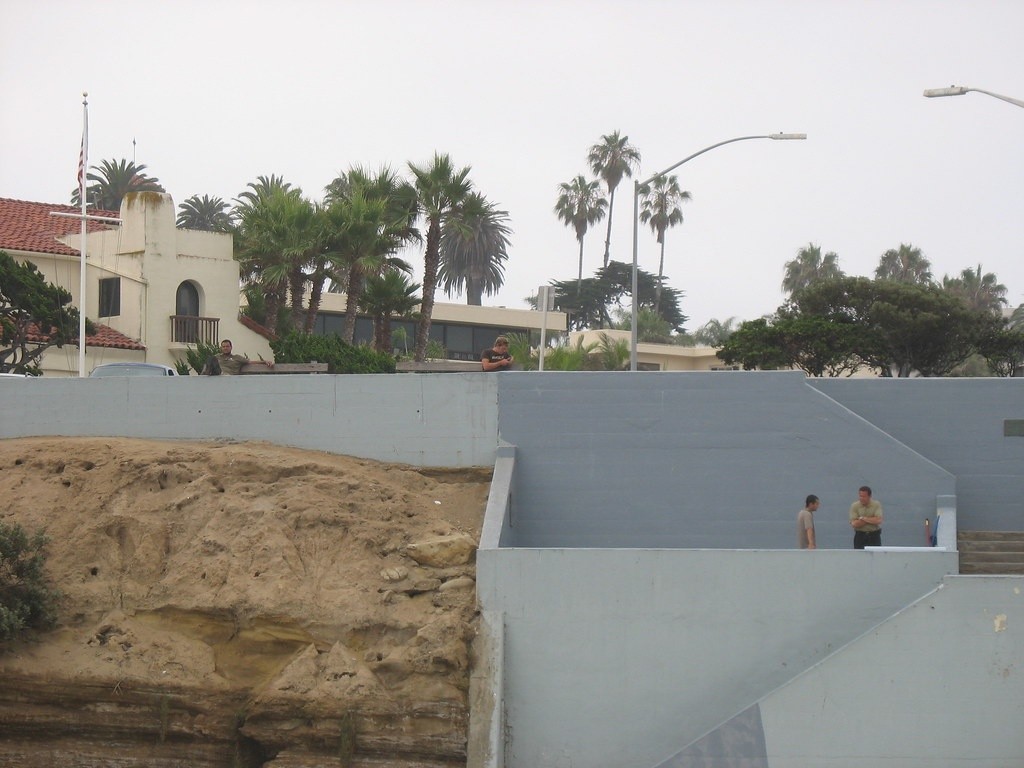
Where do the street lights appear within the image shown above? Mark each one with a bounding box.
[631,131,807,371]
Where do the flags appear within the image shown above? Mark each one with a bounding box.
[76,131,88,199]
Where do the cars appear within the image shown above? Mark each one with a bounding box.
[88,362,179,377]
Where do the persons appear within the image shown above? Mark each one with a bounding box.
[796,494,819,549]
[200,339,274,376]
[849,485,884,548]
[479,336,514,372]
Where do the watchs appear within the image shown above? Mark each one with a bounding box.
[860,517,863,520]
